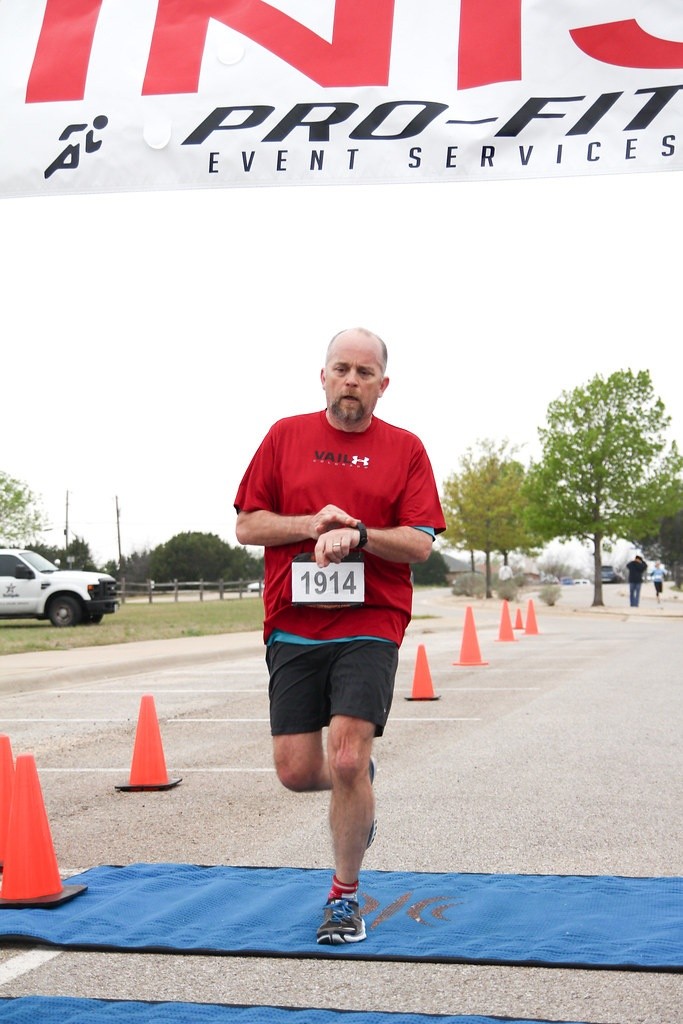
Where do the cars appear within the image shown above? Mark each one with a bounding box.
[543,565,625,585]
[246,579,264,593]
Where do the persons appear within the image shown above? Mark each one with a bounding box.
[649,559,668,598]
[233,328,447,940]
[626,555,647,607]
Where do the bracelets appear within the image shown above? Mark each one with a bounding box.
[354,523,368,550]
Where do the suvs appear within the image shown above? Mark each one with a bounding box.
[0,549,120,627]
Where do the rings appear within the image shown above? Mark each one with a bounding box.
[333,543,341,546]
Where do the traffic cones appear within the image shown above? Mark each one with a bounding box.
[114,694,183,791]
[1,736,89,907]
[405,644,441,701]
[452,605,489,665]
[512,607,525,629]
[495,599,519,641]
[522,599,541,636]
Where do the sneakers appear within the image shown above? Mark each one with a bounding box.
[315,899,367,945]
[365,755,375,849]
